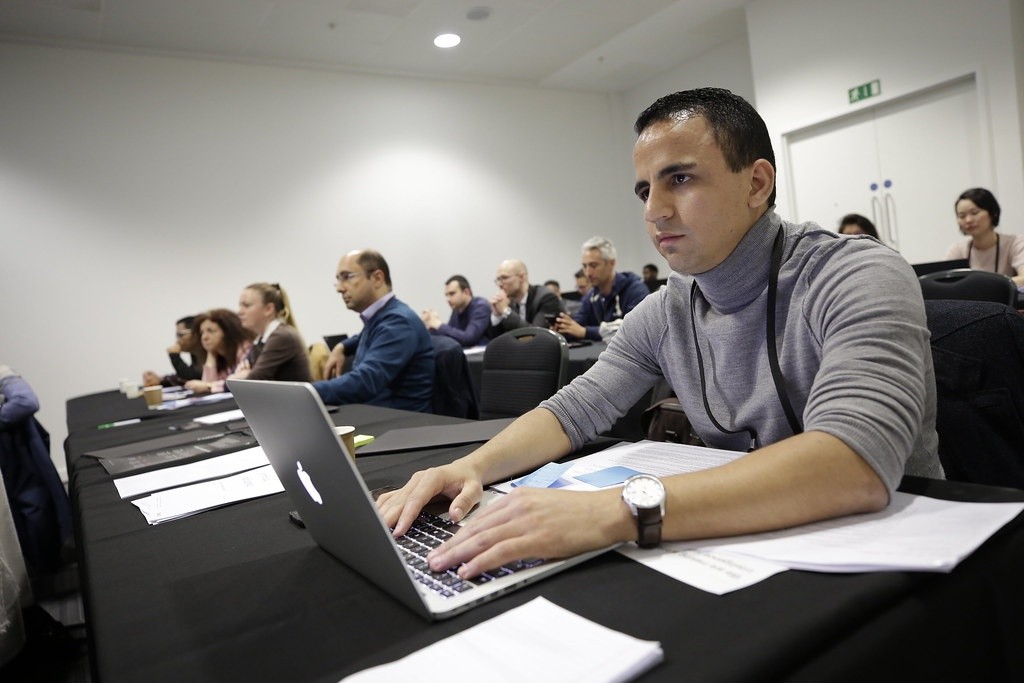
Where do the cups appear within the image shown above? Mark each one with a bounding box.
[336,426,355,466]
[144,387,162,410]
[118,378,139,400]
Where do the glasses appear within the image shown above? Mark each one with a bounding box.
[495,275,518,283]
[176,329,192,337]
[336,271,371,282]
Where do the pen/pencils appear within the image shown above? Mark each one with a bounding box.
[98,419,141,429]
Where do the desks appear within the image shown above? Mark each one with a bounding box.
[67,388,1023,683]
[462,343,608,405]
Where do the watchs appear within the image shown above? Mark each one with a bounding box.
[621,474,667,549]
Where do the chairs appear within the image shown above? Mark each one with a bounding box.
[480,327,567,418]
[916,268,1017,307]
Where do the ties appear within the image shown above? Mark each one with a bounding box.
[514,302,520,315]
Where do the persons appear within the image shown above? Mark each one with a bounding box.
[184,282,314,395]
[143,308,257,387]
[377,85,947,578]
[948,188,1024,288]
[0,365,84,604]
[312,249,434,414]
[422,213,881,339]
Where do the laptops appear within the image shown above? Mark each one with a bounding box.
[225,378,628,619]
